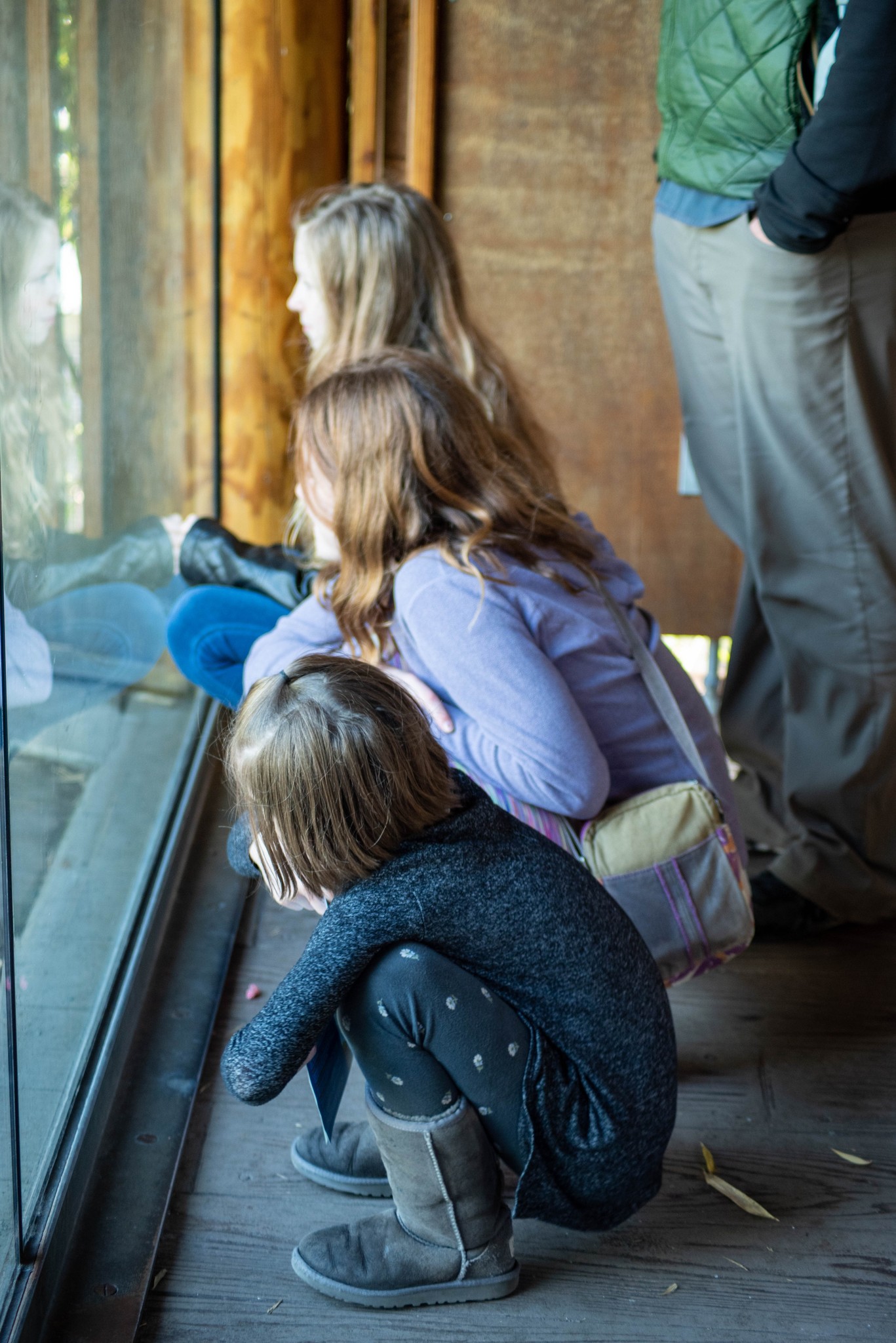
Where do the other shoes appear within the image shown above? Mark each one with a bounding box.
[747,864,874,947]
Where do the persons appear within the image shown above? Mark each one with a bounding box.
[649,0,896,959]
[165,183,605,879]
[224,348,744,1033]
[1,200,190,726]
[195,635,683,1306]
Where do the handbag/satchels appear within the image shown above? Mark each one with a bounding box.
[572,780,754,989]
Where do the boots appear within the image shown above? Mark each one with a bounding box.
[290,1091,522,1307]
[290,1118,405,1201]
